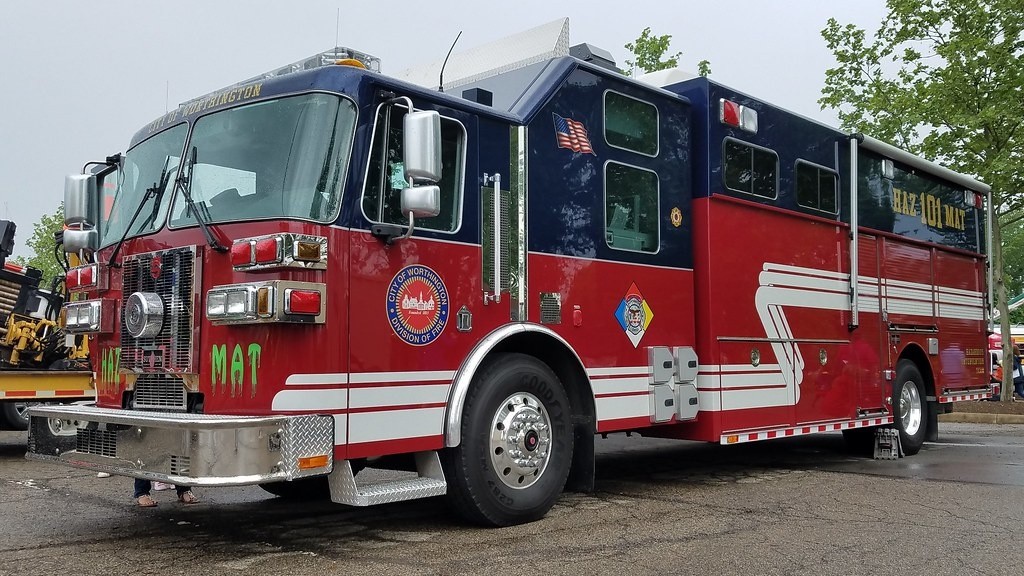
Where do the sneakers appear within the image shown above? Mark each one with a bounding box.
[155,482,166,490]
[165,483,176,489]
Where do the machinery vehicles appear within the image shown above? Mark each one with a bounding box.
[0,219,203,458]
[986,294,1024,401]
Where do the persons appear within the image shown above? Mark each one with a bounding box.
[98,472,201,507]
[988,337,1024,401]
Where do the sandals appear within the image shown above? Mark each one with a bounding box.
[135,494,159,507]
[178,491,201,503]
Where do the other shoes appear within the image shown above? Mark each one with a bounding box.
[97,472,111,477]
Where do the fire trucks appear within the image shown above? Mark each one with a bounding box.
[24,13,998,531]
